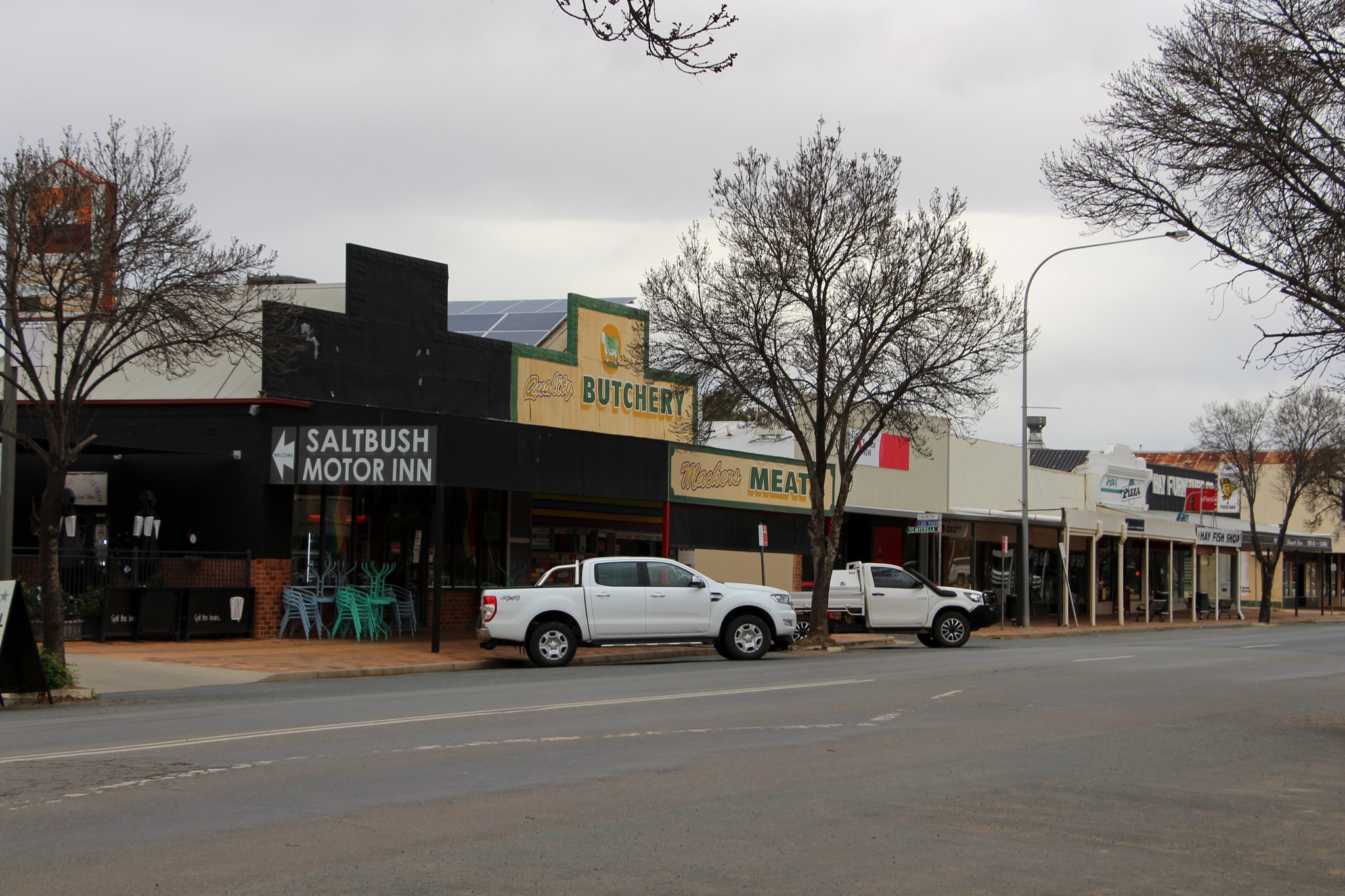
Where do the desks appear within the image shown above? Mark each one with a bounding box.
[304,559,357,640]
[360,560,396,640]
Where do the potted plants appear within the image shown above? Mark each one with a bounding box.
[18,573,112,641]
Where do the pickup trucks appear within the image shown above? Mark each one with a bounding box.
[477,555,798,668]
[789,560,998,649]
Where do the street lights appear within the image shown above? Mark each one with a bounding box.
[1021,230,1193,626]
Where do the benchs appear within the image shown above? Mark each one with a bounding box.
[1135,599,1168,622]
[1206,598,1234,620]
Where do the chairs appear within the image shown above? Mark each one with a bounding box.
[383,584,417,637]
[278,585,323,640]
[332,583,379,641]
[475,582,502,630]
